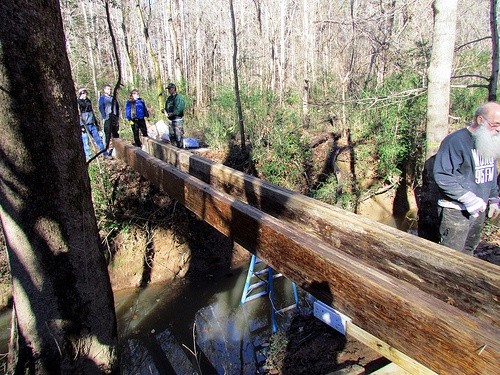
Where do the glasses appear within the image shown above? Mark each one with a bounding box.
[480,113,500,128]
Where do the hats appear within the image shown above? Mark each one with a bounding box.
[79,87,86,94]
[165,83,175,89]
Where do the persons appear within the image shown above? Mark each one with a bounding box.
[99,85,120,159]
[161,83,185,148]
[126,89,149,147]
[77,87,113,159]
[434,102,500,255]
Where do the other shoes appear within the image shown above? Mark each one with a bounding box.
[104,154,113,160]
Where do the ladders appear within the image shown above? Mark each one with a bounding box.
[240,254,299,334]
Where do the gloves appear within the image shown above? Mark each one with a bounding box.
[129,120,134,125]
[146,117,151,121]
[167,112,175,119]
[488,203,500,221]
[161,108,166,114]
[95,119,98,126]
[459,190,486,218]
[80,125,86,133]
